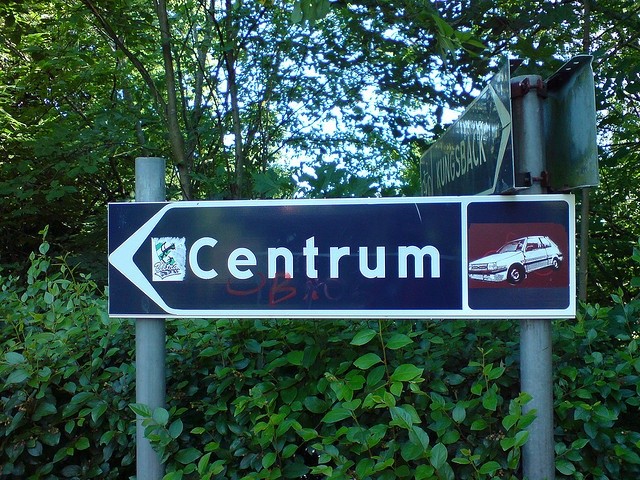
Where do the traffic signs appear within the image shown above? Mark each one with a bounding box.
[420,56,530,195]
[107,194,578,320]
[541,55,601,193]
